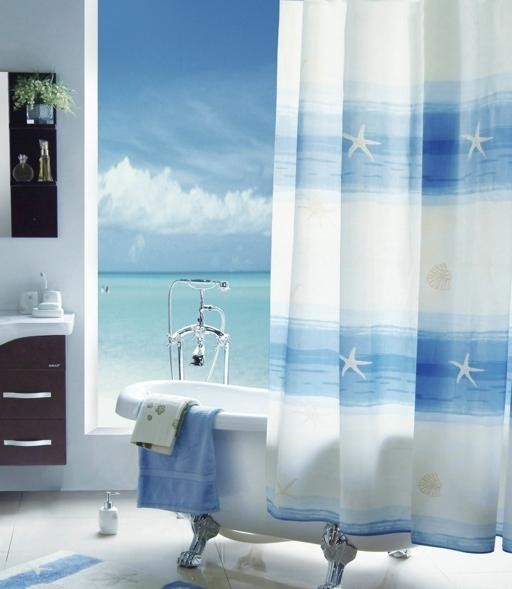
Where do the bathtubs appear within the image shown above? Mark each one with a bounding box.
[114,379,417,589]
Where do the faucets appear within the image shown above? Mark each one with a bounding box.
[191,346,205,367]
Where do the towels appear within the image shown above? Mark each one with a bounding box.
[136,400,224,515]
[129,393,201,456]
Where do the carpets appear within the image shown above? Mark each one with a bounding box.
[0,549,210,589]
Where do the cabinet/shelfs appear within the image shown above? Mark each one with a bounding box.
[0,335,67,466]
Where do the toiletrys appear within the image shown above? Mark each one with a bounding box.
[11,153,35,183]
[99,492,120,535]
[35,136,55,183]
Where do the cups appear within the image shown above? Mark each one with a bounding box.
[20,290,63,318]
